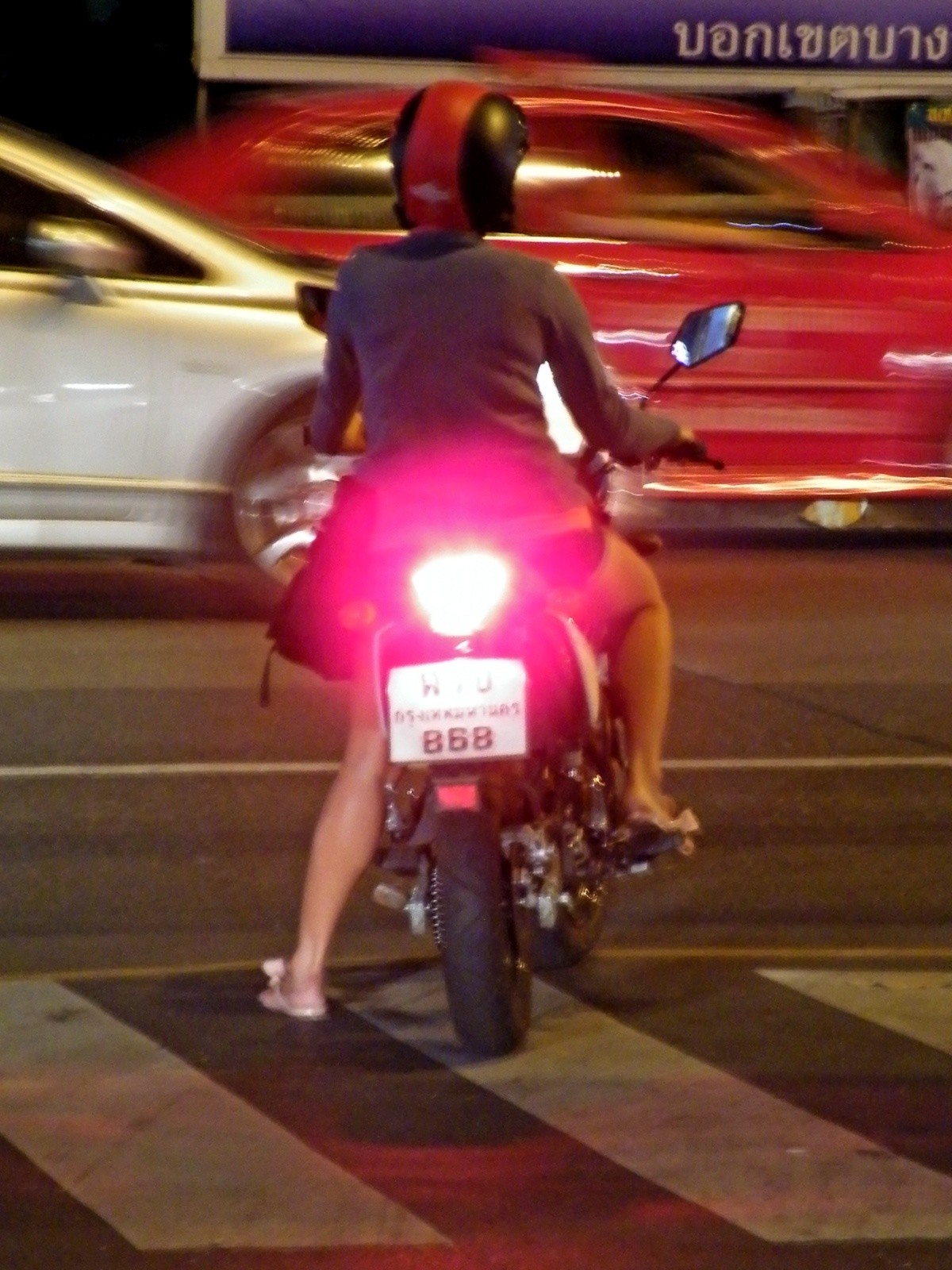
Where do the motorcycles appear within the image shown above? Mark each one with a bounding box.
[265,281,746,1061]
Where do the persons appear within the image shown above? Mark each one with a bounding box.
[251,79,703,1021]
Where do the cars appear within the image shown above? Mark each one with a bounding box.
[108,88,952,540]
[0,125,605,609]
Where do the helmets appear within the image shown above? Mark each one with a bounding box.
[390,77,530,235]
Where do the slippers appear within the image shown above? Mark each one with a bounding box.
[622,787,684,831]
[257,959,326,1018]
